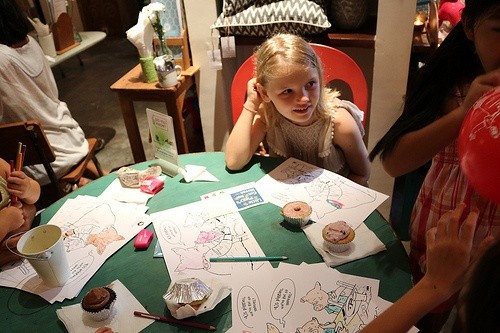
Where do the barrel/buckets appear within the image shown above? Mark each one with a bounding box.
[6,224,71,286]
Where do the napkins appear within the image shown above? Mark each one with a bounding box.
[127,12,155,59]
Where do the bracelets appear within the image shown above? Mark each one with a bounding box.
[242,105,258,114]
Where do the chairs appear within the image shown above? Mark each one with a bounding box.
[230,44,367,130]
[0,120,104,195]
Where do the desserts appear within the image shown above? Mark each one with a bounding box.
[280,201,312,227]
[81,286,117,320]
[163,278,212,306]
[322,221,355,252]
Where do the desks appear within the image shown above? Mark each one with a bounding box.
[0,153,414,333]
[110,62,193,166]
[50,31,106,68]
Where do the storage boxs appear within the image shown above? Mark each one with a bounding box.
[151,0,190,71]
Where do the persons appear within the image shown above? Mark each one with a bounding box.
[0,0,90,190]
[0,158,40,243]
[355,0,500,333]
[224,32,372,187]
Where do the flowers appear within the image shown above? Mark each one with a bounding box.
[144,2,163,39]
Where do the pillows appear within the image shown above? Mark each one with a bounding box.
[211,0,331,36]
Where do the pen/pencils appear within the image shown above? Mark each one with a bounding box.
[209,256,289,262]
[133,311,216,331]
[10,141,27,205]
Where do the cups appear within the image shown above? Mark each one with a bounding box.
[414,0,429,32]
[37,32,57,58]
[138,56,157,82]
[155,65,182,87]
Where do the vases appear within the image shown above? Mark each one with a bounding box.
[158,38,172,56]
